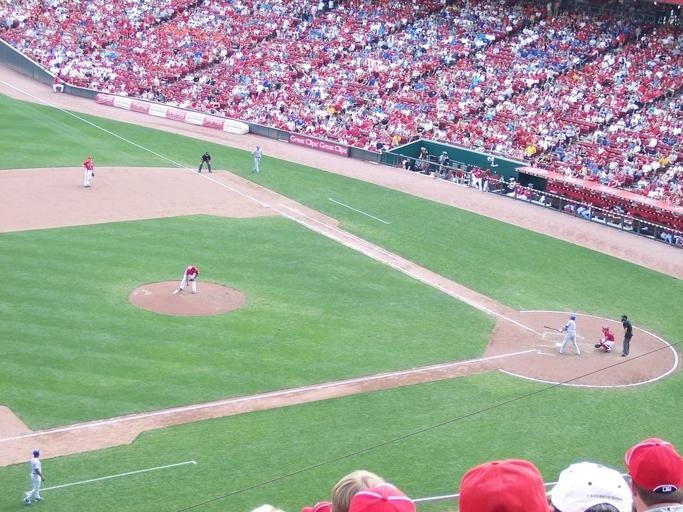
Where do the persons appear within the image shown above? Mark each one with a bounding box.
[620,315,633,357]
[560,315,581,355]
[301,471,416,512]
[24,448,45,504]
[625,438,683,512]
[1,1,683,251]
[599,326,615,352]
[174,266,198,294]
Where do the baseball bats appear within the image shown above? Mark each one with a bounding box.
[544,326,560,332]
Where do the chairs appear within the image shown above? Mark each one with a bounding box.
[1,1,683,227]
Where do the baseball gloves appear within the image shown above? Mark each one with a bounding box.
[594,342,602,348]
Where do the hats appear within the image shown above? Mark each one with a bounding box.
[348,482,414,511]
[624,437,683,492]
